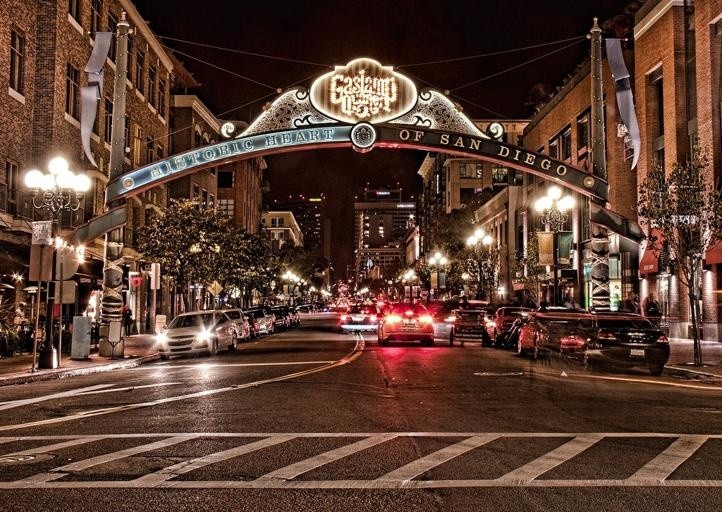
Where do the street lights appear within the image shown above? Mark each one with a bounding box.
[428,253,446,298]
[467,228,494,299]
[404,269,417,305]
[282,271,297,306]
[23,158,92,370]
[533,185,574,304]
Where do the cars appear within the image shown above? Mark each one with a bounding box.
[158,306,300,361]
[482,305,537,349]
[516,306,587,361]
[649,339,654,345]
[435,309,488,345]
[340,305,378,333]
[559,311,669,376]
[376,302,434,345]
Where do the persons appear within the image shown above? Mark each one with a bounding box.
[623,290,665,330]
[122,305,133,337]
